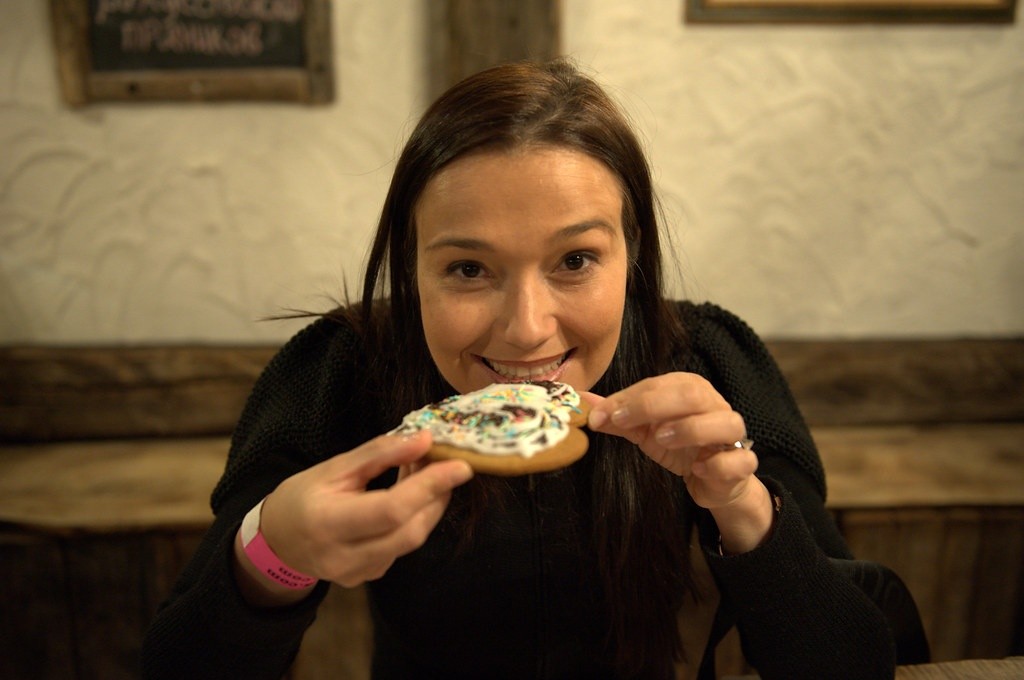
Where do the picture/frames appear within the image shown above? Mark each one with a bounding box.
[50,0,337,108]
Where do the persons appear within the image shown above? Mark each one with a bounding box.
[130,58,931,680]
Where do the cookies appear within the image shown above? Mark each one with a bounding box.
[390,378,592,474]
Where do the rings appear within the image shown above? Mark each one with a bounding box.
[720,439,754,452]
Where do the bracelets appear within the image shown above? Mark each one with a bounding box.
[239,493,318,590]
